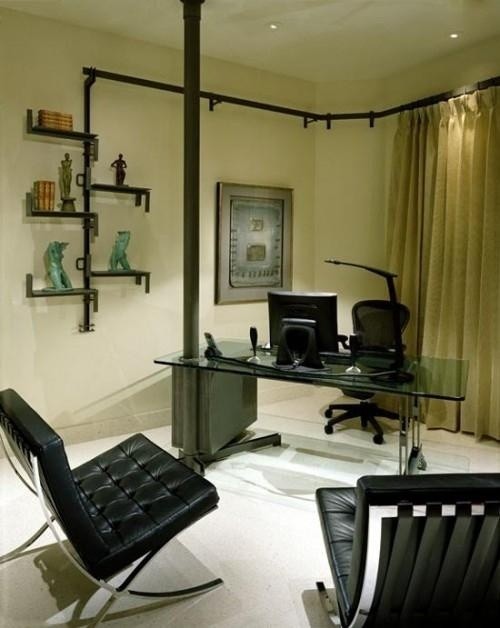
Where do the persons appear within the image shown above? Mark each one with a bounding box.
[110,230,131,272]
[42,242,72,291]
[61,152,73,196]
[110,153,127,185]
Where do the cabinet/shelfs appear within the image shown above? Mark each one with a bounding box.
[27,110,150,332]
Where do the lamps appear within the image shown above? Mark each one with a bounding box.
[322,260,405,368]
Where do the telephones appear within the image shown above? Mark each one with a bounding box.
[205,333,222,360]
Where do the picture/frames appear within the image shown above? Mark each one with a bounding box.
[216,180,293,306]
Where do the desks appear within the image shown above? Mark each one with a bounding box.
[155,340,470,476]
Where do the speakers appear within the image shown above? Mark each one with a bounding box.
[345,334,362,375]
[246,326,261,363]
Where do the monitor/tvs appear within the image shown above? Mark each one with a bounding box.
[267,290,339,373]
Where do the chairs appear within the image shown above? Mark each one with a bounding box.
[0,388,223,628]
[324,301,410,444]
[316,474,500,628]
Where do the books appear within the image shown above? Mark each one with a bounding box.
[38,108,73,131]
[34,180,55,211]
